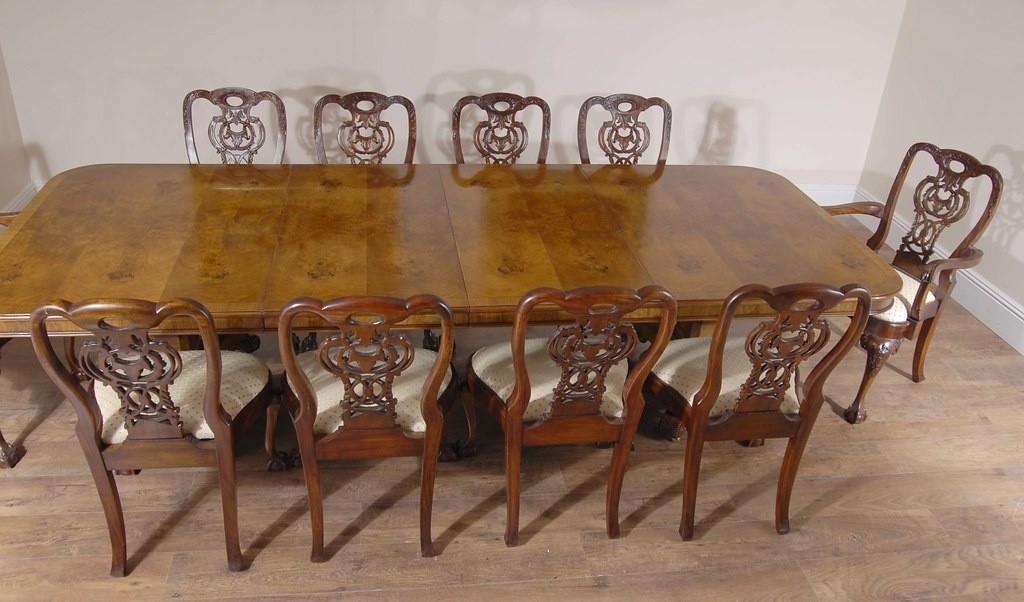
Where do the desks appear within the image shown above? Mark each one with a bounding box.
[0,166,902,452]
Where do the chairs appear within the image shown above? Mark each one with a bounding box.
[0,87,1003,574]
